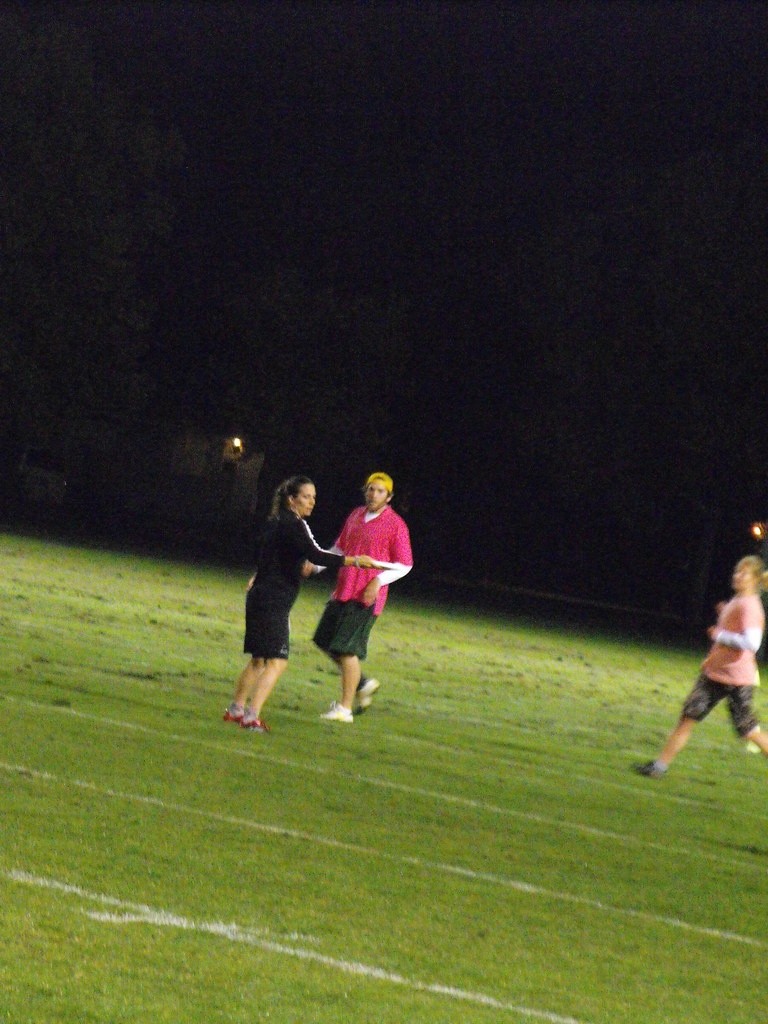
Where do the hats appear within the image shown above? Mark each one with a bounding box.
[364,471,394,495]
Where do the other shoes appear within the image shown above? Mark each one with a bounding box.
[320,701,355,723]
[631,759,667,780]
[238,715,273,732]
[351,677,381,716]
[222,707,245,724]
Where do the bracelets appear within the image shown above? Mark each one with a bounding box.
[354,555,360,569]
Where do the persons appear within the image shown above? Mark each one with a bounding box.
[224,476,372,733]
[303,472,413,725]
[629,556,768,778]
